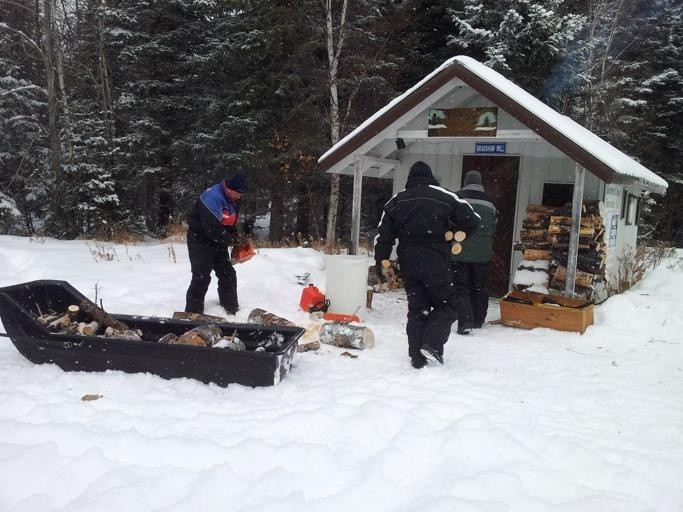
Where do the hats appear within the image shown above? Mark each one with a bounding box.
[227,174,247,194]
[463,170,482,186]
[405,160,437,189]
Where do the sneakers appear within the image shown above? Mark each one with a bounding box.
[457,328,469,335]
[419,345,444,365]
[225,307,240,315]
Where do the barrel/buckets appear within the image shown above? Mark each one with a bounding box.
[324,255,369,322]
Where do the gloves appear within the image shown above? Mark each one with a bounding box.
[233,220,254,234]
[223,231,242,247]
[376,263,401,283]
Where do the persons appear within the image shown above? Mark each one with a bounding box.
[448,167,502,337]
[370,160,482,373]
[184,171,256,320]
